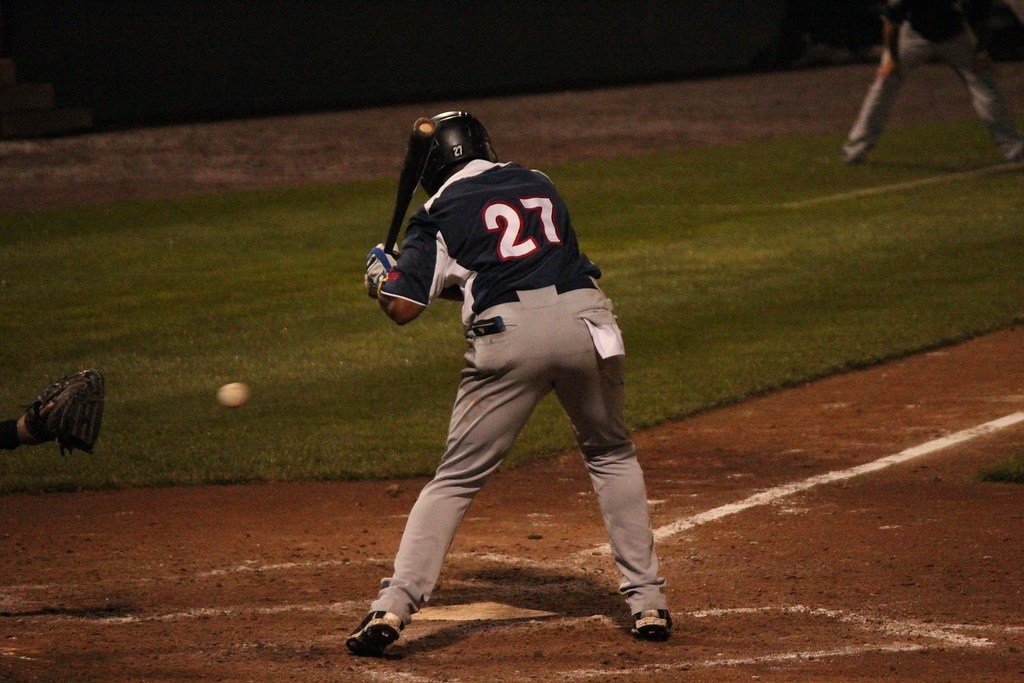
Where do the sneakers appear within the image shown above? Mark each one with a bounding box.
[631,597,672,633]
[344,613,404,657]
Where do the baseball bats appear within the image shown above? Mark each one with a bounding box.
[381,114,436,255]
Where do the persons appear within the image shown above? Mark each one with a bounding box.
[0,369,104,455]
[842,0,1024,162]
[346,111,672,655]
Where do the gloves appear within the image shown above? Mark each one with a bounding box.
[366,241,400,284]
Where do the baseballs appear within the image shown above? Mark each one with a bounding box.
[216,383,253,409]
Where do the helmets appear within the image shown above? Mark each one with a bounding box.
[420,112,496,197]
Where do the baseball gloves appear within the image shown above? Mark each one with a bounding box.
[24,367,104,451]
